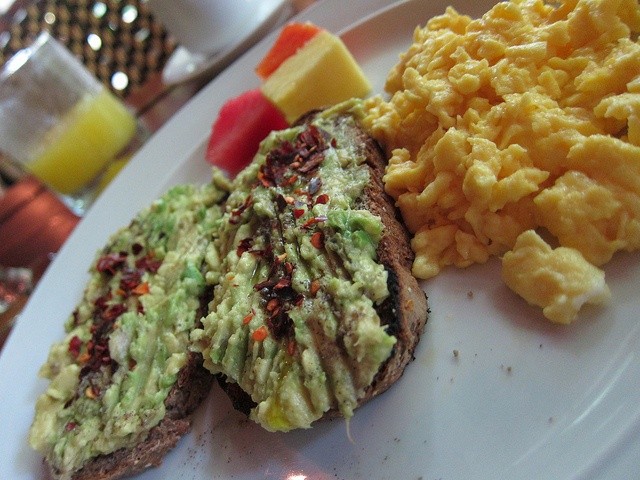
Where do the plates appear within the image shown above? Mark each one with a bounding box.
[1,0,640,479]
[162,8,293,86]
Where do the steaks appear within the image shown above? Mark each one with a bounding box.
[192,95,430,434]
[28,167,234,479]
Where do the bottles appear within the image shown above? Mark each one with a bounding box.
[0,32,151,216]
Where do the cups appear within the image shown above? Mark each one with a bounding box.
[147,0,283,57]
